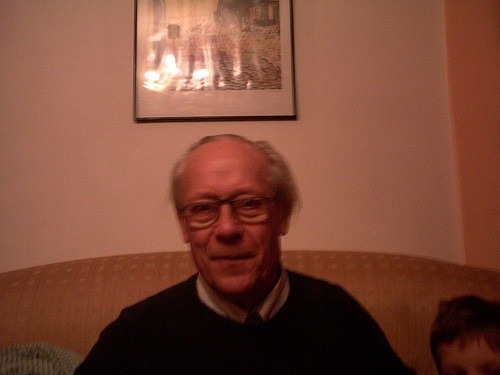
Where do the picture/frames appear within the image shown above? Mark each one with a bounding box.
[133,0,298,123]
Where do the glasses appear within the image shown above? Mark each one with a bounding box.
[179,195,279,231]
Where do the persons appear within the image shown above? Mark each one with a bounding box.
[69,133,422,375]
[430,293,500,375]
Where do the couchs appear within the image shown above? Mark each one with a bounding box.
[0,250,500,375]
